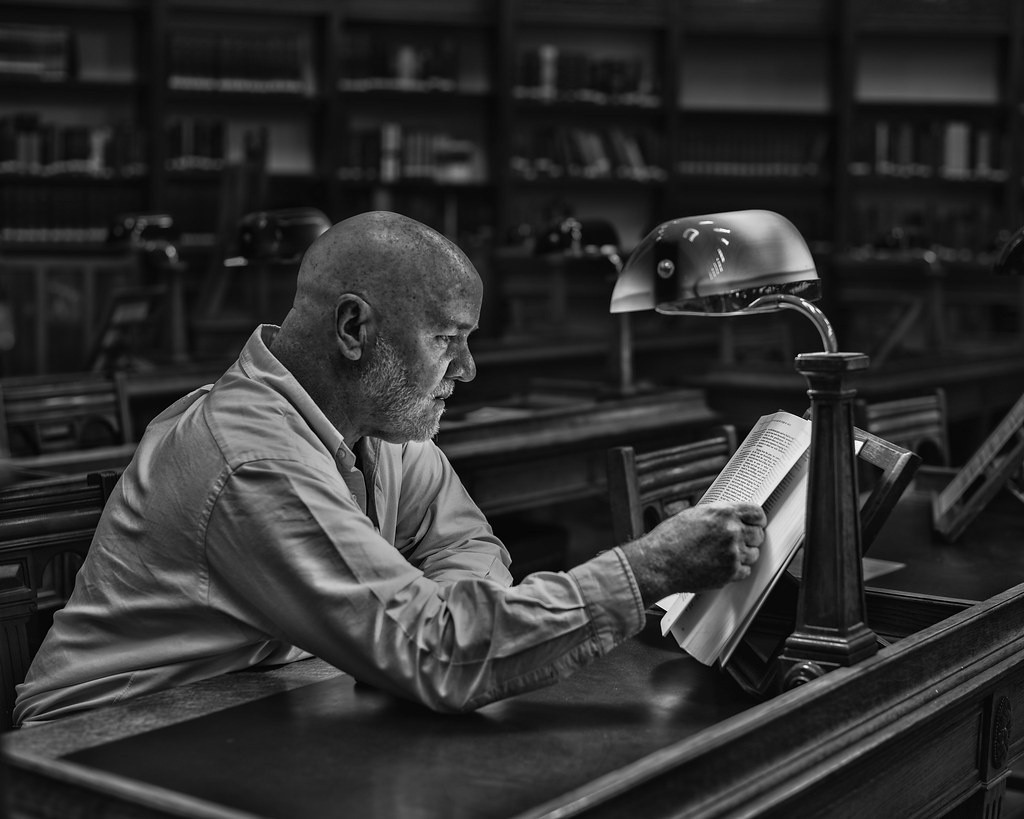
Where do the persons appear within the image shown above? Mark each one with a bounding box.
[12,211,767,732]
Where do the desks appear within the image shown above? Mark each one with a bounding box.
[0,504,1024,819]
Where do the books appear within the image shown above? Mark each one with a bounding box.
[349,119,474,182]
[853,116,1014,182]
[513,119,665,180]
[152,115,268,174]
[681,134,823,175]
[335,30,489,96]
[515,46,665,108]
[654,410,870,668]
[1,111,143,179]
[0,183,217,259]
[168,36,318,99]
[852,190,1007,266]
[1,23,81,80]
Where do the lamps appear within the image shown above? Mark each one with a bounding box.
[195,206,331,370]
[610,209,877,693]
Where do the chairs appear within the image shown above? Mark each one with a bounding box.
[0,470,118,732]
[857,388,951,468]
[609,425,738,547]
